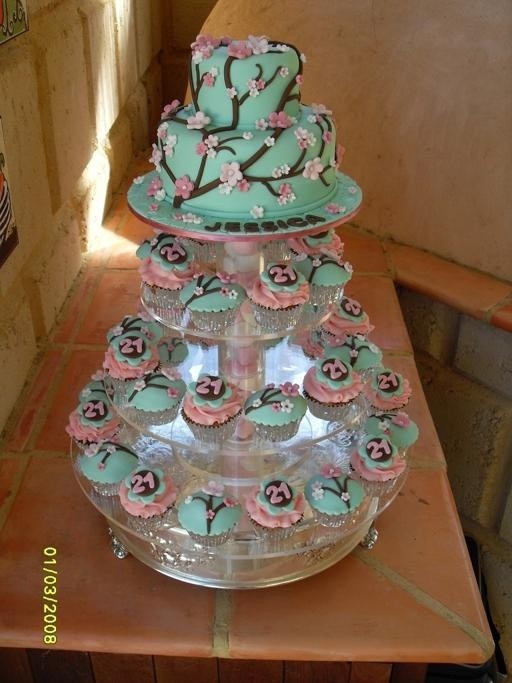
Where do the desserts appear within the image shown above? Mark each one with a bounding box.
[66,228,419,549]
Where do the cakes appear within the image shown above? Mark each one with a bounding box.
[145,33,347,221]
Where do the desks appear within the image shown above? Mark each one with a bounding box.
[1,272,503,682]
